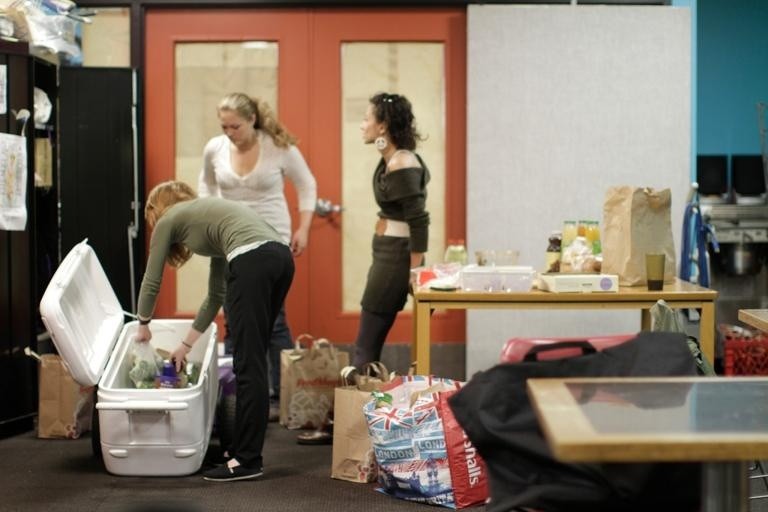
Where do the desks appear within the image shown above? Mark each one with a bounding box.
[737,307,768,335]
[525,369,767,511]
[408,281,720,385]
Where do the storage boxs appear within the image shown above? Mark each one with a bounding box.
[45,235,219,477]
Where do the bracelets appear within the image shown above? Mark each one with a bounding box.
[183,341,193,348]
[138,314,151,325]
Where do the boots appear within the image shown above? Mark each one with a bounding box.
[296,411,335,445]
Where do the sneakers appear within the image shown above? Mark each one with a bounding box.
[206,449,232,464]
[202,457,264,482]
[267,398,280,422]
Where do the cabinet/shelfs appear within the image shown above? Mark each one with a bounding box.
[1,44,135,449]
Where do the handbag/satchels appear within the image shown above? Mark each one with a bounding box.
[37,353,94,439]
[330,360,494,510]
[279,333,350,431]
[447,331,706,512]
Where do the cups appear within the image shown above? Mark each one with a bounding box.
[645,252,666,291]
[473,248,523,294]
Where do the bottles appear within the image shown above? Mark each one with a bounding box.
[562,219,601,259]
[163,359,176,378]
[545,237,561,271]
[444,238,467,266]
[369,390,394,406]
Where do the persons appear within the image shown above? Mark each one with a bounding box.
[136,181,295,480]
[196,94,318,419]
[298,94,431,445]
